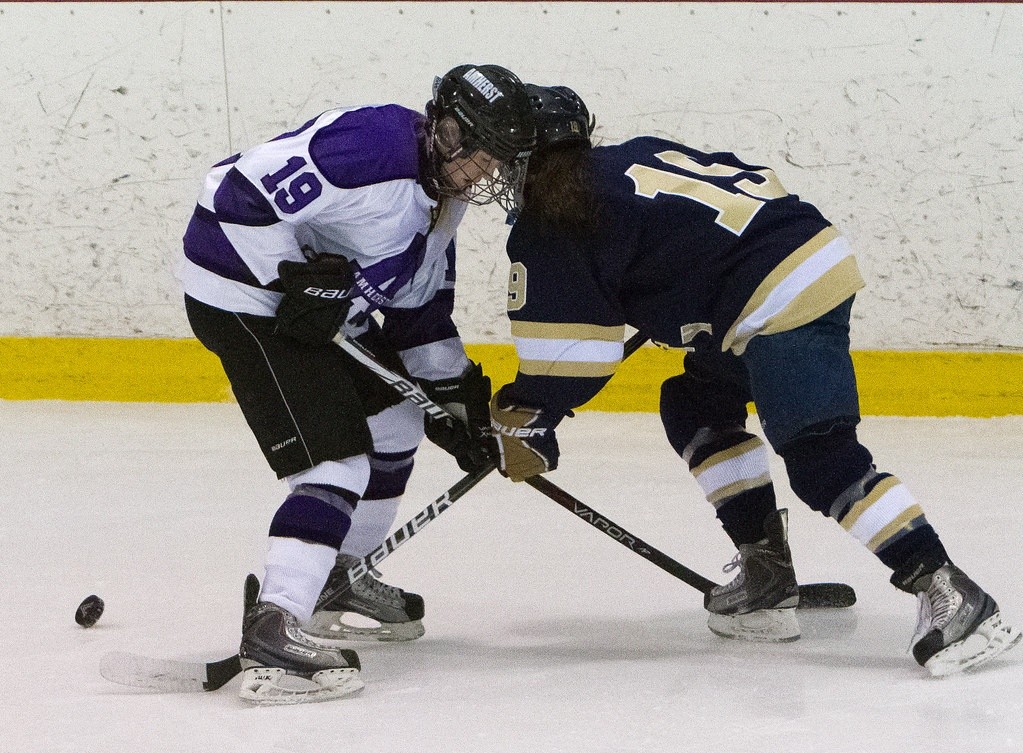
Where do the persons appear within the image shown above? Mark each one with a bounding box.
[182,64,531,704]
[492,87,1022,680]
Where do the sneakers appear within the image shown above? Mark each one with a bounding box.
[705,506,802,643]
[238,573,365,705]
[304,552,426,645]
[890,548,1022,678]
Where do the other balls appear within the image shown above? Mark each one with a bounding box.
[74,594,105,628]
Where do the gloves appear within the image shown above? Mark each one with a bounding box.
[486,383,575,483]
[274,253,356,353]
[420,358,497,472]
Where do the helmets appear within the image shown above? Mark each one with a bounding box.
[488,83,591,216]
[422,64,528,203]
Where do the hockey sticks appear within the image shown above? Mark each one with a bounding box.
[99,458,498,693]
[328,327,857,611]
